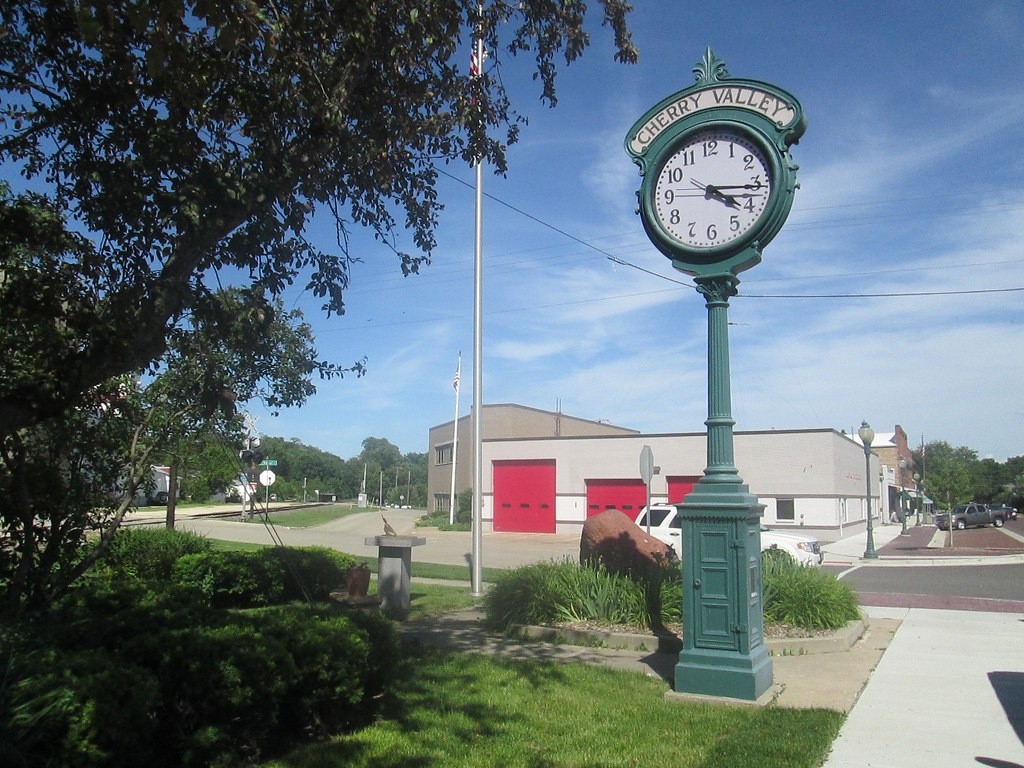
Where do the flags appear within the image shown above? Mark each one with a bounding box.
[469,2,488,117]
[452,354,460,395]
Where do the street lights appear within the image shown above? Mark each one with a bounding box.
[898,454,910,536]
[914,470,922,526]
[856,419,878,560]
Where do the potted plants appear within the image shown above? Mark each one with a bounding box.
[345,560,372,601]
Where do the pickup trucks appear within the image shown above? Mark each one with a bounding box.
[934,502,1006,531]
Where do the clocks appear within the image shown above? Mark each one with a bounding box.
[652,131,772,248]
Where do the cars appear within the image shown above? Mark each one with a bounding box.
[1001,506,1018,521]
